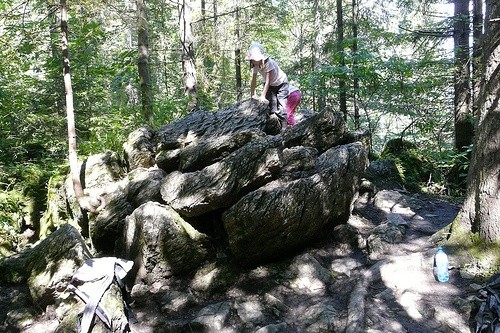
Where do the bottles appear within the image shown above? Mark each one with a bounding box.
[435,246,449,282]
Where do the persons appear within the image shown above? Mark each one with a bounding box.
[245,43,301,130]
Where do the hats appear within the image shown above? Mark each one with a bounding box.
[244,42,271,61]
